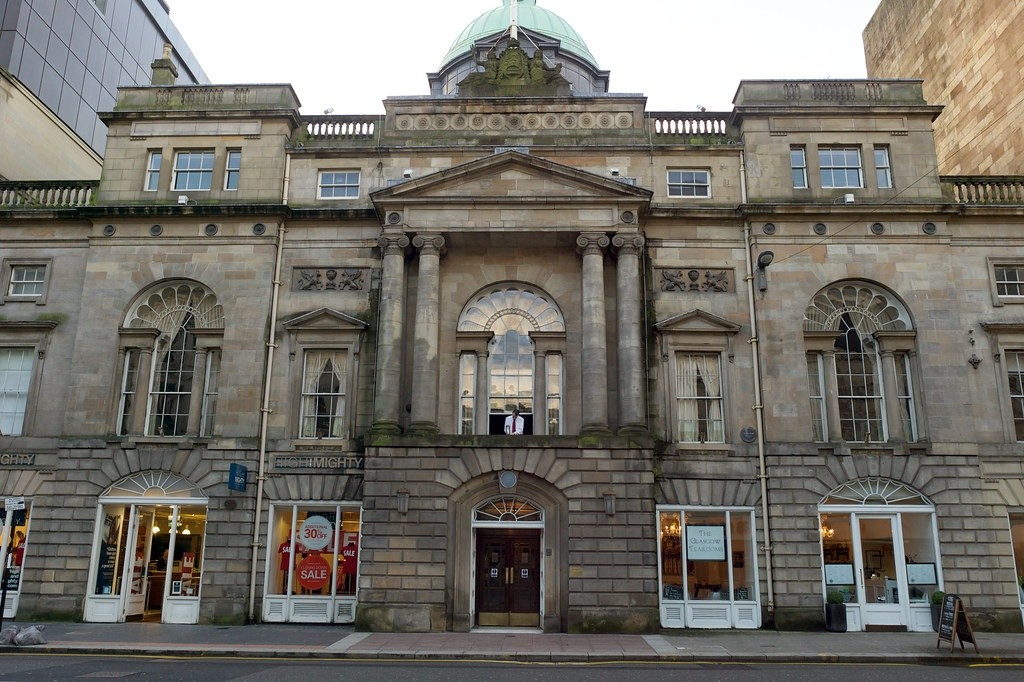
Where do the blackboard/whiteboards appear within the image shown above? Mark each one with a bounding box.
[938,593,976,642]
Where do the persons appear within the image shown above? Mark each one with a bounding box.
[156,550,168,571]
[456,44,500,86]
[504,409,524,435]
[528,49,563,83]
